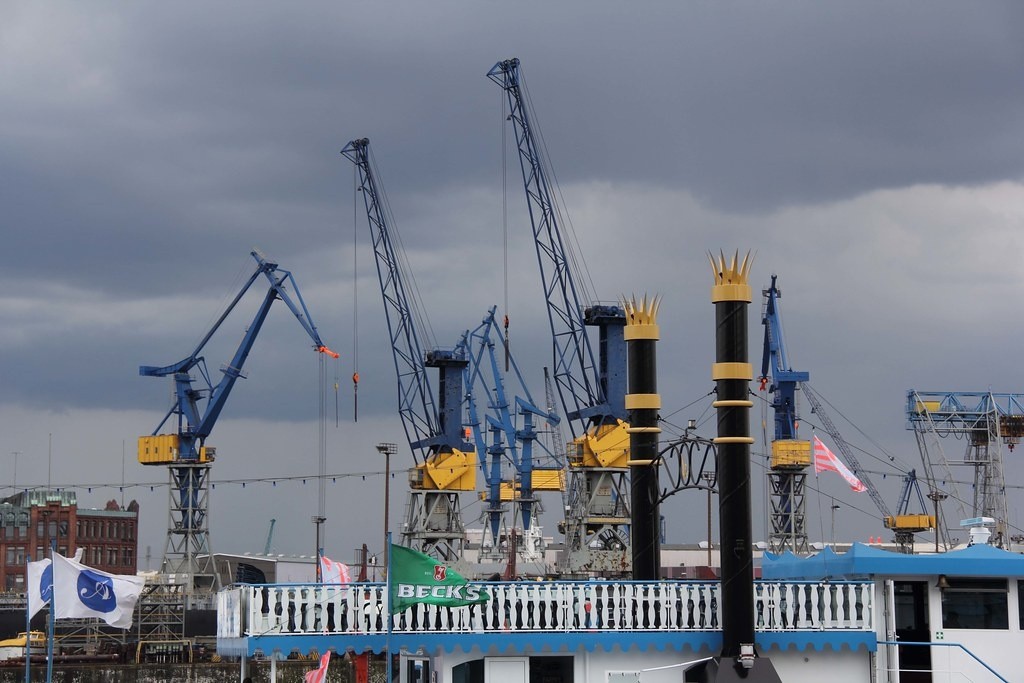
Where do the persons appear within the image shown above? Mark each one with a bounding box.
[995,532,1004,549]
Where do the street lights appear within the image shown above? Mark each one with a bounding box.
[374,442,398,584]
[311,514,327,585]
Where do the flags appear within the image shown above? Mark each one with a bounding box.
[389,545,490,615]
[814,435,868,493]
[305,650,331,683]
[51,551,146,629]
[319,554,350,588]
[28,548,84,620]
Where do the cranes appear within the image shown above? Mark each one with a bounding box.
[758,274,940,558]
[903,388,1023,558]
[132,252,347,607]
[340,60,630,585]
